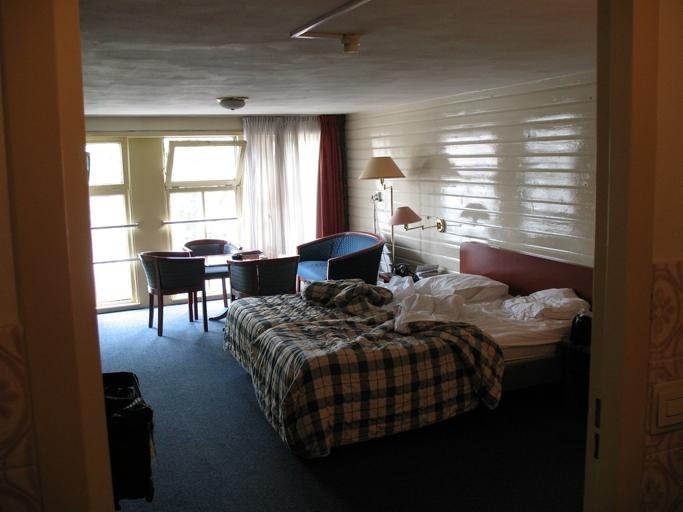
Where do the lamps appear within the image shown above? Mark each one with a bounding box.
[389,207,444,232]
[217,97,247,110]
[359,156,405,272]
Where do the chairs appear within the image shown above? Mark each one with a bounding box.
[183,239,238,321]
[296,232,385,295]
[227,256,300,301]
[138,251,207,336]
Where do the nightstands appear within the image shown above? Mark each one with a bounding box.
[379,272,392,282]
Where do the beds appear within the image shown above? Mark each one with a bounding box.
[223,242,593,465]
